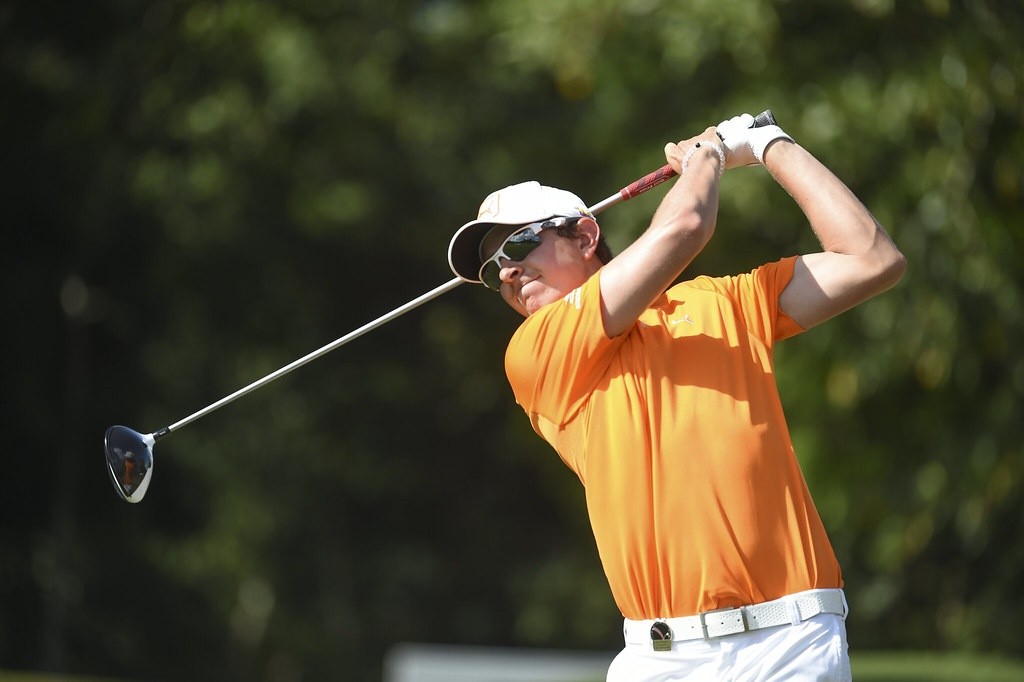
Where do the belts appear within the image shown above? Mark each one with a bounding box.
[623,590,846,645]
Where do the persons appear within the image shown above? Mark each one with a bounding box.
[448,114,908,681]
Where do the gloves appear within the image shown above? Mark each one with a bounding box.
[716,112,794,168]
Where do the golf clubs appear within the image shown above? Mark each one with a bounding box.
[103,107,779,505]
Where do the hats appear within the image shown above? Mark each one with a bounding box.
[448,181,597,284]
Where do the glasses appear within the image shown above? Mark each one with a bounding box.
[479,216,583,291]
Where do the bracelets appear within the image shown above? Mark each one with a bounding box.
[681,140,726,179]
